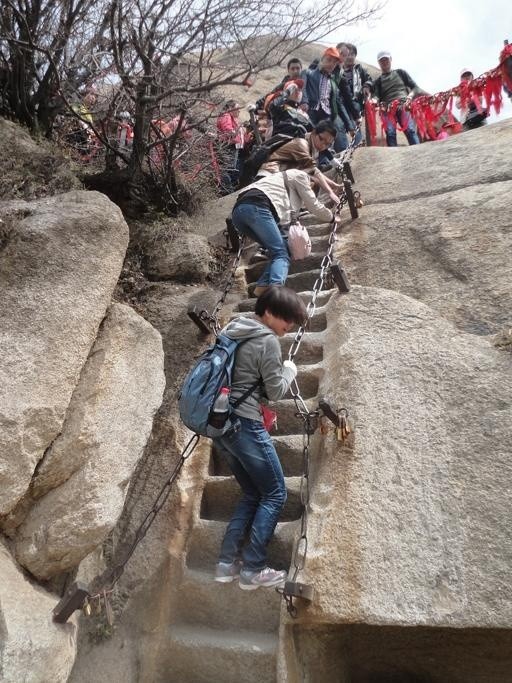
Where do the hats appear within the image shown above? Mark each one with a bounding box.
[459,68,472,78]
[324,47,343,64]
[264,91,282,109]
[376,52,391,62]
[284,79,304,91]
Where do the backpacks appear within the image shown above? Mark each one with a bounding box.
[260,133,314,164]
[179,335,269,439]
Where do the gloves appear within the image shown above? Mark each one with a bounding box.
[330,158,344,169]
[283,359,297,375]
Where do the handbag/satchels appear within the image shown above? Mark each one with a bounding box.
[259,399,278,431]
[288,220,312,261]
[462,109,490,128]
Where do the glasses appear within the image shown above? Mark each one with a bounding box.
[317,133,332,149]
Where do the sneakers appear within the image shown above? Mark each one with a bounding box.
[254,248,269,262]
[214,558,244,583]
[238,567,287,590]
[253,286,270,298]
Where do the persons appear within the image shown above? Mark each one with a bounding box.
[253,120,343,262]
[247,42,372,170]
[436,121,457,140]
[208,285,310,592]
[455,67,491,132]
[232,170,341,295]
[217,99,244,196]
[371,51,421,147]
[169,111,193,171]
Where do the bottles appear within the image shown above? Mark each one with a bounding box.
[203,385,231,438]
